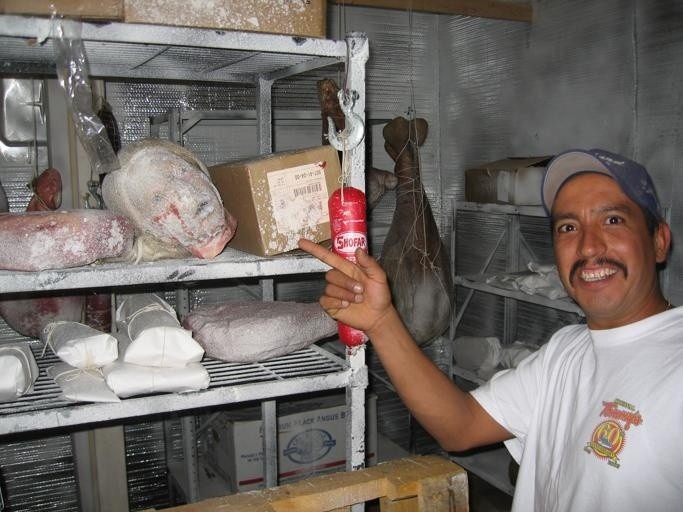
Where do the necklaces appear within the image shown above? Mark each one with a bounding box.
[665,302,671,310]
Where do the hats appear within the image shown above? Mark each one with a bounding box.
[537,147,666,224]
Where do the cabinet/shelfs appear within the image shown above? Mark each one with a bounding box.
[0,0,364,512]
[153,104,426,503]
[442,192,673,501]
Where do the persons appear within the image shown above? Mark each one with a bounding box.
[295,145,683,511]
[94,138,240,267]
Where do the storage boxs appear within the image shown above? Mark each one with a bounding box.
[195,386,378,497]
[465,155,555,210]
[211,145,344,260]
